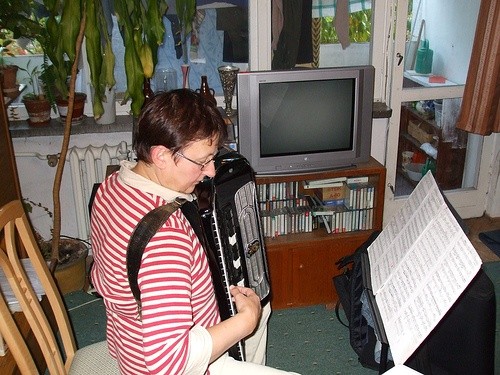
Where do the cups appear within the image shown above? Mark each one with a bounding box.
[155,68,176,90]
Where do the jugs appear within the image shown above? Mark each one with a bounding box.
[415,39,432,74]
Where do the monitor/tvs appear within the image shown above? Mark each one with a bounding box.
[237,65,375,177]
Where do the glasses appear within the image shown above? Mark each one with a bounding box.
[173,150,215,171]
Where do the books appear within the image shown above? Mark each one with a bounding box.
[254,175,379,210]
[256,209,372,238]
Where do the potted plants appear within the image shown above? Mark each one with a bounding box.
[14,58,58,126]
[0,0,196,295]
[46,61,88,126]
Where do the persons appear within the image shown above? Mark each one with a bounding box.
[86,87,303,375]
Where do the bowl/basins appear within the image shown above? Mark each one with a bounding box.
[405,163,427,180]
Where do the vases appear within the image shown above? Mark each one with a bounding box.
[0,58,20,93]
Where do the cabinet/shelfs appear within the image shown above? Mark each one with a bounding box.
[251,157,387,310]
[397,76,469,191]
[1,94,60,375]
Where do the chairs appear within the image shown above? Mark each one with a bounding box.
[0,199,121,375]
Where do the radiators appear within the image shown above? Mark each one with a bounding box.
[47,141,139,249]
